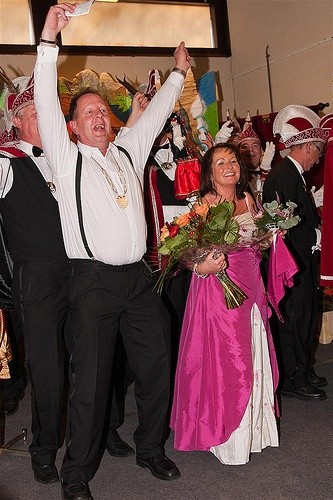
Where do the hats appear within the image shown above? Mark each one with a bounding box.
[1,67,38,122]
[273,106,326,149]
[234,110,262,149]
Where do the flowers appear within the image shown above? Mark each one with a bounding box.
[252,191,302,242]
[152,191,248,311]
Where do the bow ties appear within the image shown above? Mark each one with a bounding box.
[153,143,170,152]
[31,146,45,157]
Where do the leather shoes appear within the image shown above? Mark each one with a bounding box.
[30,456,59,484]
[301,368,327,386]
[6,389,25,416]
[137,450,179,481]
[60,480,94,500]
[282,378,325,400]
[106,429,135,457]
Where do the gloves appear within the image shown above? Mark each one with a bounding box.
[260,142,275,171]
[213,120,232,145]
[312,185,324,207]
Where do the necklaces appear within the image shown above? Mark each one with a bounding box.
[151,148,173,171]
[88,148,133,210]
[30,155,56,195]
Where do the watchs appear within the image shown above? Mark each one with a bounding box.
[39,37,59,45]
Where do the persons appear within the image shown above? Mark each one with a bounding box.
[1,84,135,484]
[212,110,278,208]
[143,125,196,412]
[168,141,289,465]
[260,104,328,403]
[31,3,193,500]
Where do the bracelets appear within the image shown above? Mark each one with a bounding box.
[192,263,209,279]
[172,67,187,79]
